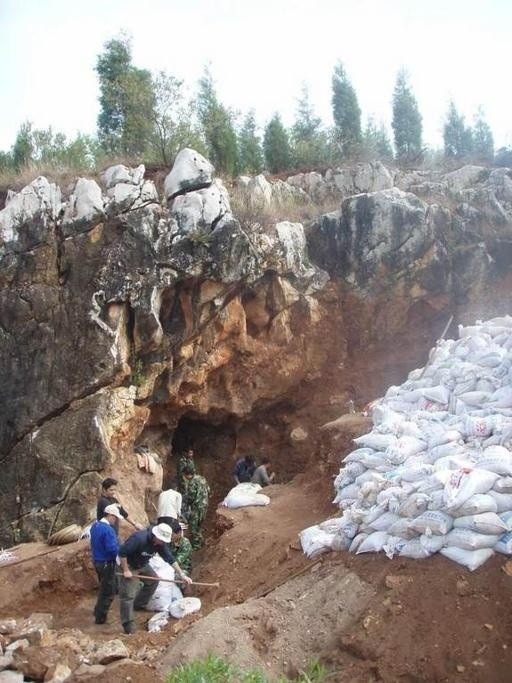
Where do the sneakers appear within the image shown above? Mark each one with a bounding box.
[124,621,134,633]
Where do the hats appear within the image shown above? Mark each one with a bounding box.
[105,505,124,520]
[152,524,172,544]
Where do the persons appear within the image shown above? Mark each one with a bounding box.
[250,458,278,486]
[231,454,256,484]
[89,444,212,635]
